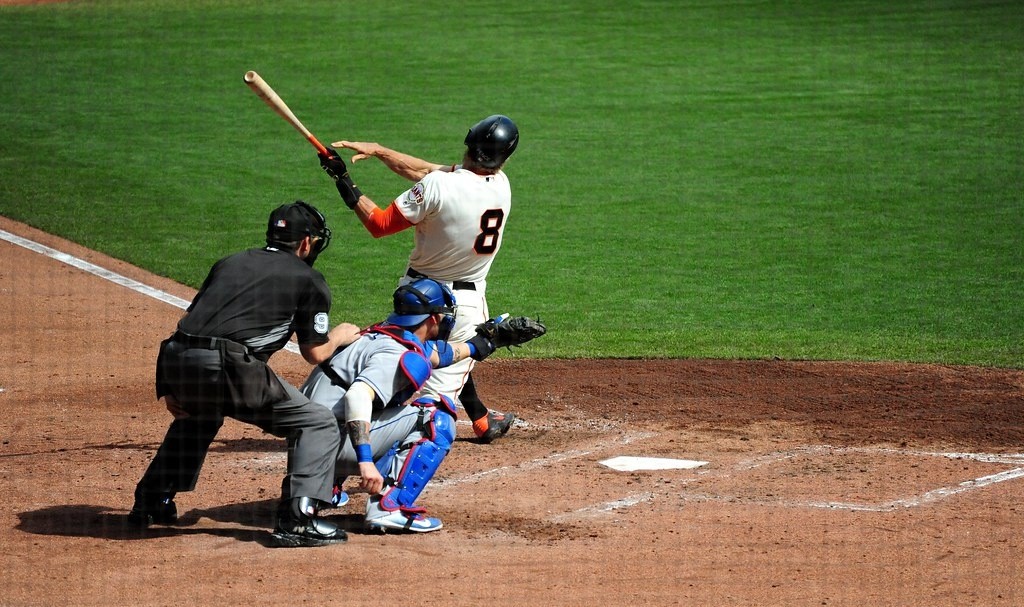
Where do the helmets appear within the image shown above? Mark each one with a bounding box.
[466,115,520,169]
[385,280,445,327]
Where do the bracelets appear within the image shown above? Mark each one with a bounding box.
[354,444,372,463]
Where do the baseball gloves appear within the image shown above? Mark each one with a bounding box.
[476,315,546,348]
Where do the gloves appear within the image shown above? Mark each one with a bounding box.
[318,144,362,208]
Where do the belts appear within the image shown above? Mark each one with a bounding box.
[174,329,245,353]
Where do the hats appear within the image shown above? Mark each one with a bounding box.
[268,203,321,242]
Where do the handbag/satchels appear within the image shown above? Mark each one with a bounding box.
[221,351,291,410]
[155,333,187,401]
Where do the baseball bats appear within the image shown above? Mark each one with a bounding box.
[244,69,327,160]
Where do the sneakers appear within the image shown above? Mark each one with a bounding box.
[127,490,178,525]
[271,513,348,547]
[363,501,443,534]
[473,405,515,442]
[316,483,348,512]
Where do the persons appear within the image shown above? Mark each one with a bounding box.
[290,278,546,533]
[129,201,361,546]
[318,115,519,443]
